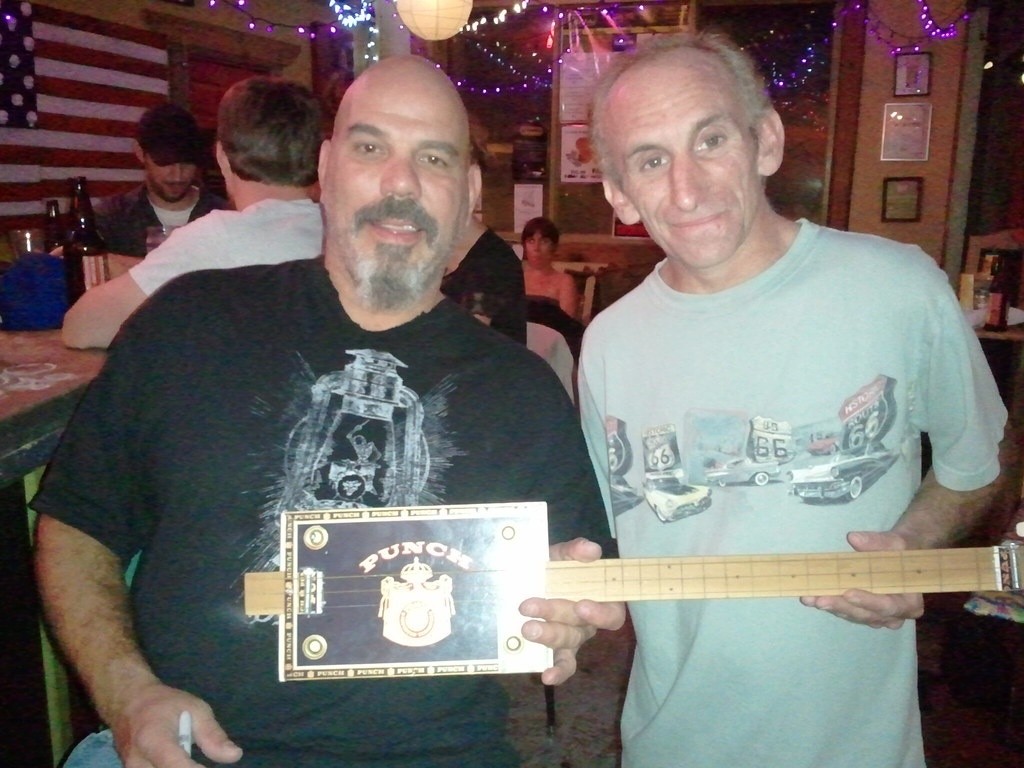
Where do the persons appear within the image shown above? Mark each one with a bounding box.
[54,76,580,353]
[575,27,1012,768]
[32,52,631,768]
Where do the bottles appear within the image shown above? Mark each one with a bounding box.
[984,260,1014,332]
[62,177,111,307]
[42,200,65,258]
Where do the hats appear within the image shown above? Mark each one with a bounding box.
[138,103,202,167]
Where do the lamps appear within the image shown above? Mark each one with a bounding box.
[396,0,473,41]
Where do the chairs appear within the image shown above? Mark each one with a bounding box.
[551,260,609,328]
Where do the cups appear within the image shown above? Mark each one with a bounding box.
[147,226,180,253]
[12,230,45,259]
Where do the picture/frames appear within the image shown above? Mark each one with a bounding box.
[879,103,932,162]
[880,176,923,224]
[894,52,932,97]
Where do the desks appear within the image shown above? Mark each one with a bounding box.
[972,324,1024,425]
[0,327,111,767]
